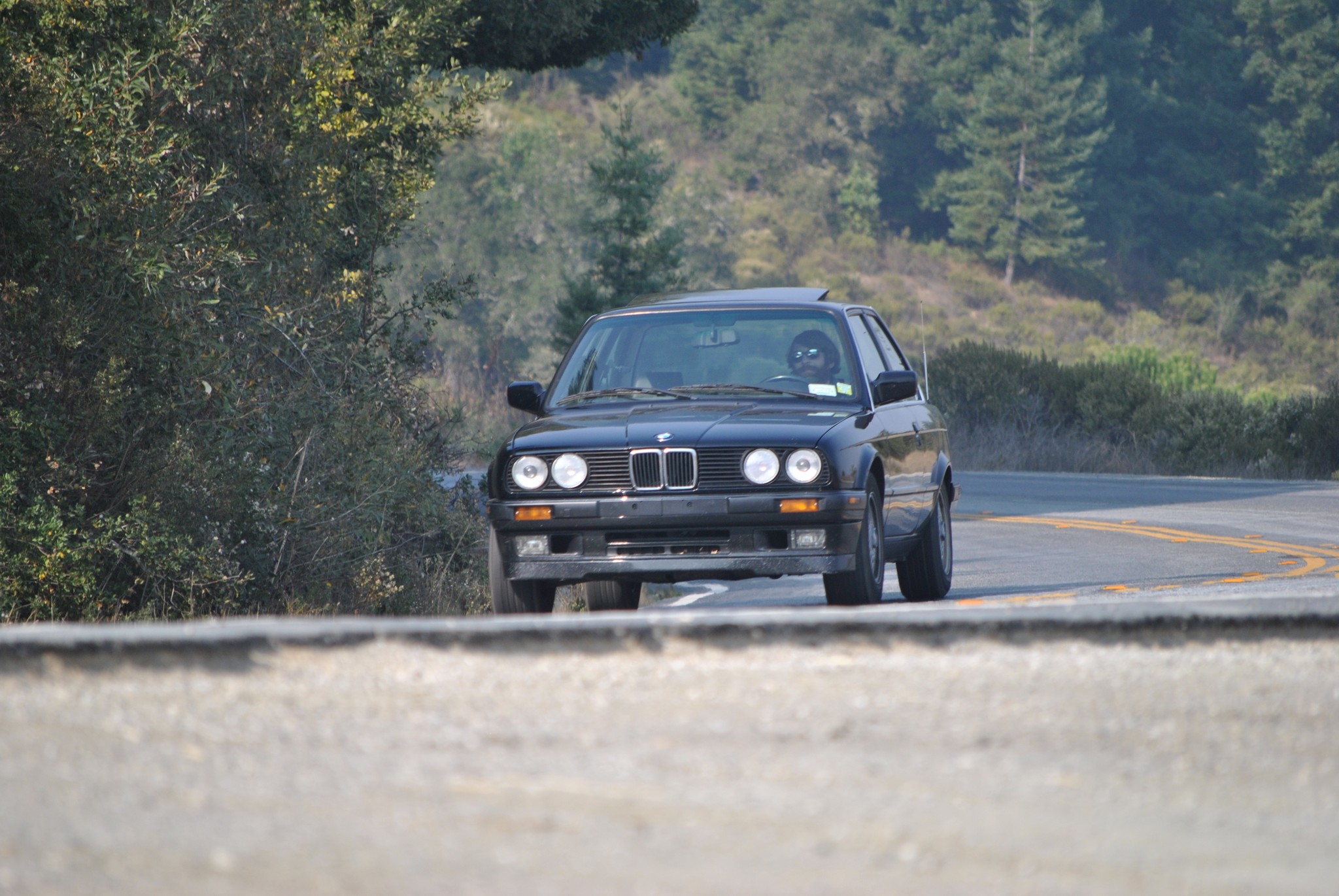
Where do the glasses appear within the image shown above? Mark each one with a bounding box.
[790,348,822,362]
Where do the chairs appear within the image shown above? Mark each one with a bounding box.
[628,372,684,401]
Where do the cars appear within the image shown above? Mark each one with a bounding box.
[484,284,956,612]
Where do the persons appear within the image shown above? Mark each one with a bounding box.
[787,328,847,397]
